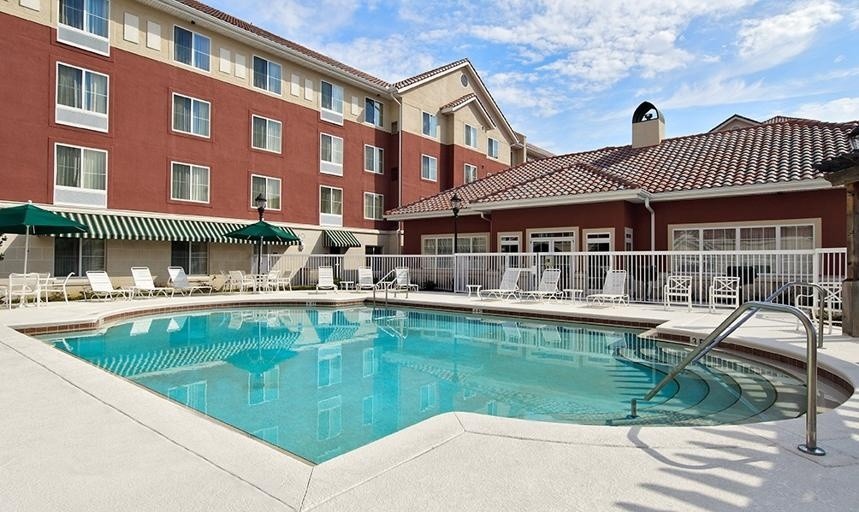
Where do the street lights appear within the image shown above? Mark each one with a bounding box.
[254,323,264,402]
[451,336,460,391]
[450,193,462,255]
[255,192,266,283]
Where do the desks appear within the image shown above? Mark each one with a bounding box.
[466,283,482,298]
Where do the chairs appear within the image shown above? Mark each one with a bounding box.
[482,267,633,310]
[0,266,297,307]
[130,307,411,336]
[316,264,419,293]
[709,276,741,312]
[795,283,843,336]
[663,275,693,313]
[461,316,637,351]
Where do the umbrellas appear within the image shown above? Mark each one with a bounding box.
[224,219,300,282]
[0,204,90,304]
